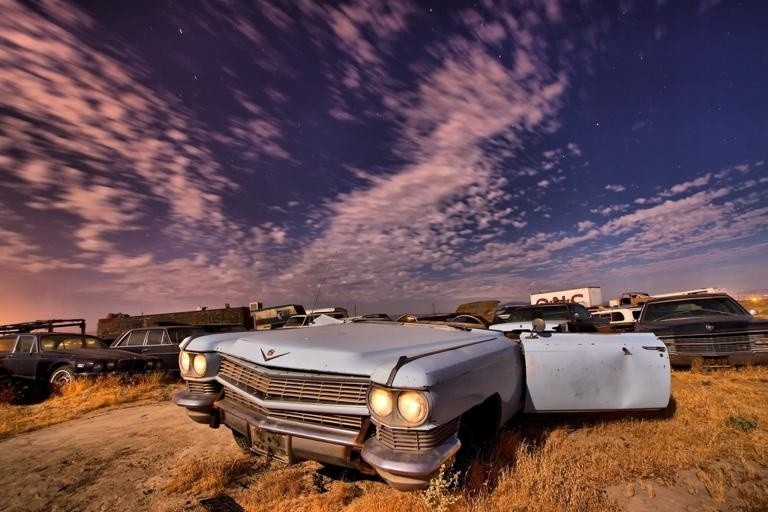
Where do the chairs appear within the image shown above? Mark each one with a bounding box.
[457,301,500,324]
